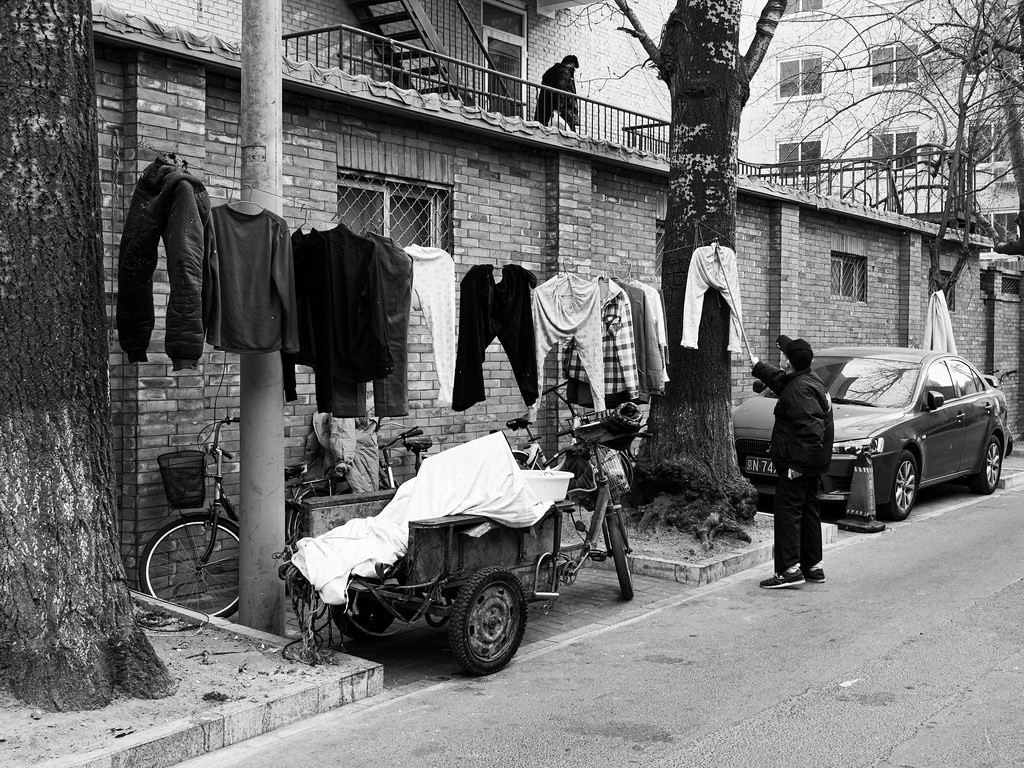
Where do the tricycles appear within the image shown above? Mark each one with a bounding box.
[281,399,646,681]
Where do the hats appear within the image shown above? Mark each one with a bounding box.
[776,335,813,370]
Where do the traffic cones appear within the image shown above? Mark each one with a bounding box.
[834,445,888,534]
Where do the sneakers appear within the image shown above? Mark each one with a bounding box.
[802,568,825,583]
[760,568,806,588]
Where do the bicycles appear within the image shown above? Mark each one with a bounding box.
[982,369,1019,459]
[134,414,337,622]
[335,424,441,492]
[508,379,634,501]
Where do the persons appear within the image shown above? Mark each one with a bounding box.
[749,335,835,589]
[532,55,582,134]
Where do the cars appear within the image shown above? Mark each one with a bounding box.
[725,341,1009,521]
